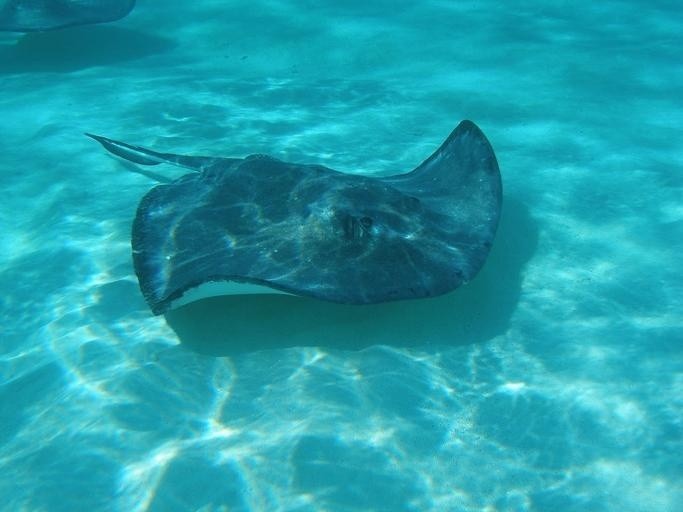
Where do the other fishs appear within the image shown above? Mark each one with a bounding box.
[83,120,503,318]
